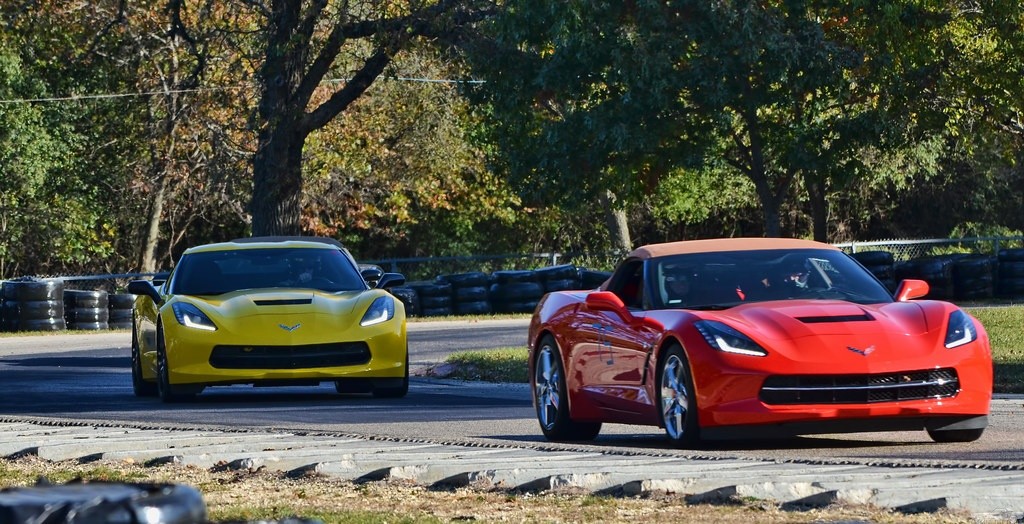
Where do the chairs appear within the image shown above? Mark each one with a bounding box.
[687,269,734,305]
[189,262,225,293]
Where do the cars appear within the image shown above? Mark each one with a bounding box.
[127,236,411,404]
[529,237,993,443]
